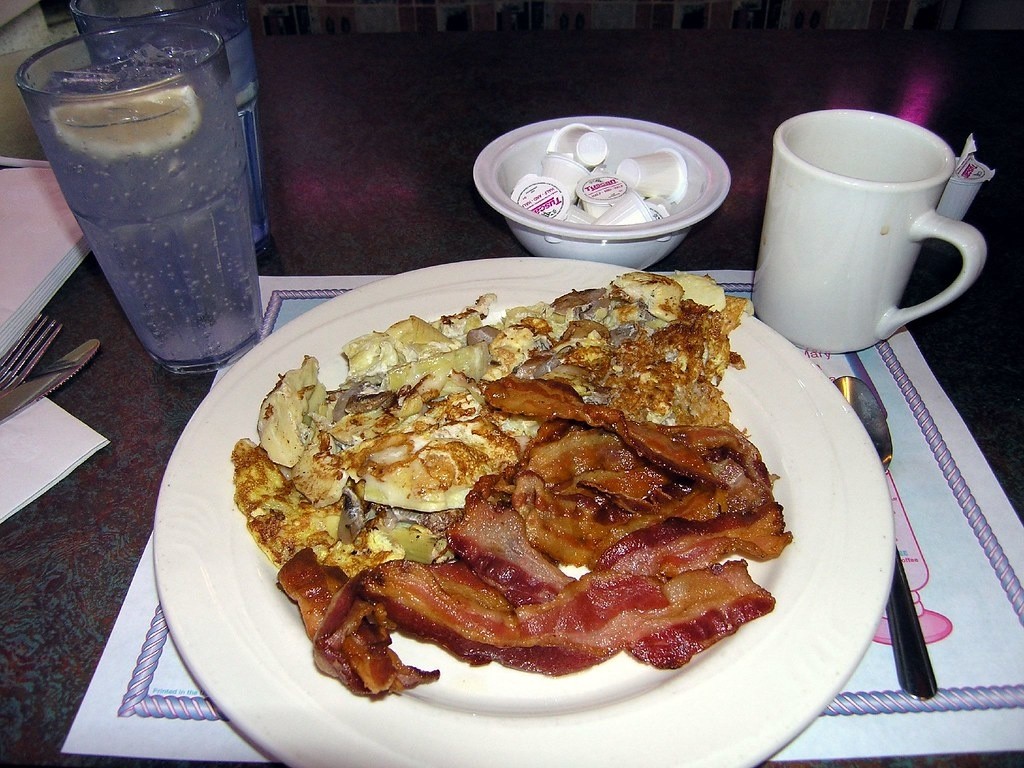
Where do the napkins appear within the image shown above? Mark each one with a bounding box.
[0,367,111,526]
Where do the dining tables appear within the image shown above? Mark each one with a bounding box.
[0,30,1023,768]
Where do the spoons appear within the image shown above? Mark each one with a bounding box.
[834,376,939,701]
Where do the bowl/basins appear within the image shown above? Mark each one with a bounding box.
[474,116,732,270]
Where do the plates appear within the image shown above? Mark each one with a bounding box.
[153,258,897,768]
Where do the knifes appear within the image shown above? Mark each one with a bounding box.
[0,339,101,422]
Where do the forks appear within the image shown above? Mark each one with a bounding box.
[1,312,63,394]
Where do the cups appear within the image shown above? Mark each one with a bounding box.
[14,22,263,374]
[69,0,268,255]
[752,108,987,353]
